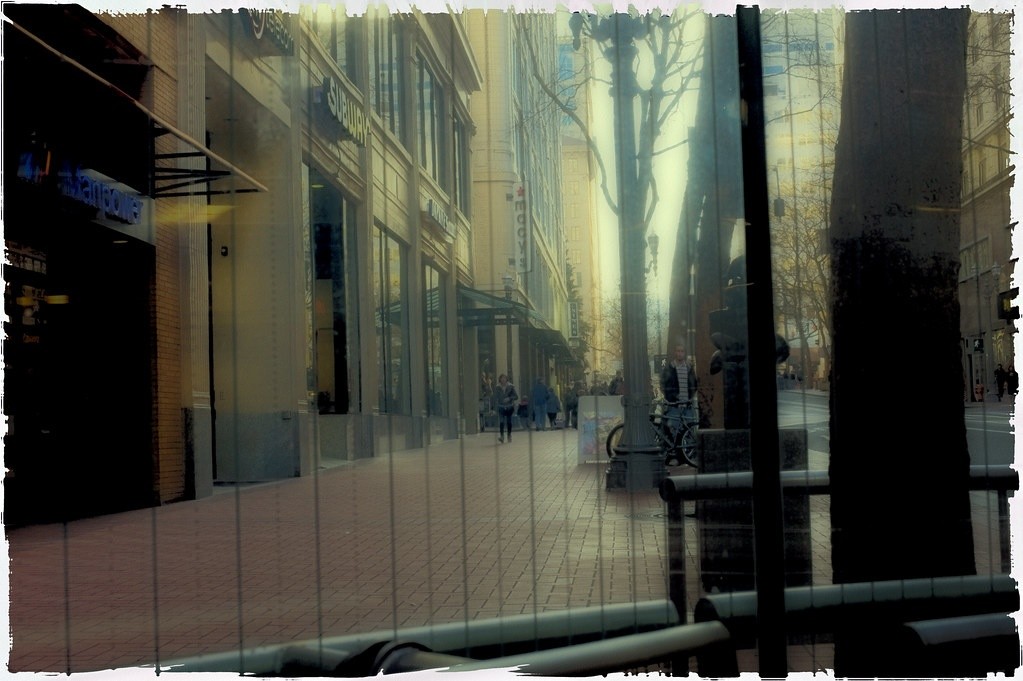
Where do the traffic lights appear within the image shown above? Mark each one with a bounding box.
[974,338,985,351]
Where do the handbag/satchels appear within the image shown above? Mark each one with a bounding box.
[497,405,514,417]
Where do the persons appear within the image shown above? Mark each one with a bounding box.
[659,343,698,454]
[490,374,518,443]
[609,370,624,395]
[994,364,1019,402]
[517,377,587,432]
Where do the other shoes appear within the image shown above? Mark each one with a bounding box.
[498,438,504,443]
[508,436,512,442]
[669,459,677,465]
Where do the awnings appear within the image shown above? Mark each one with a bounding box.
[532,328,576,362]
[375,286,440,328]
[459,286,546,324]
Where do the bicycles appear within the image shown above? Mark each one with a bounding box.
[606,396,701,468]
